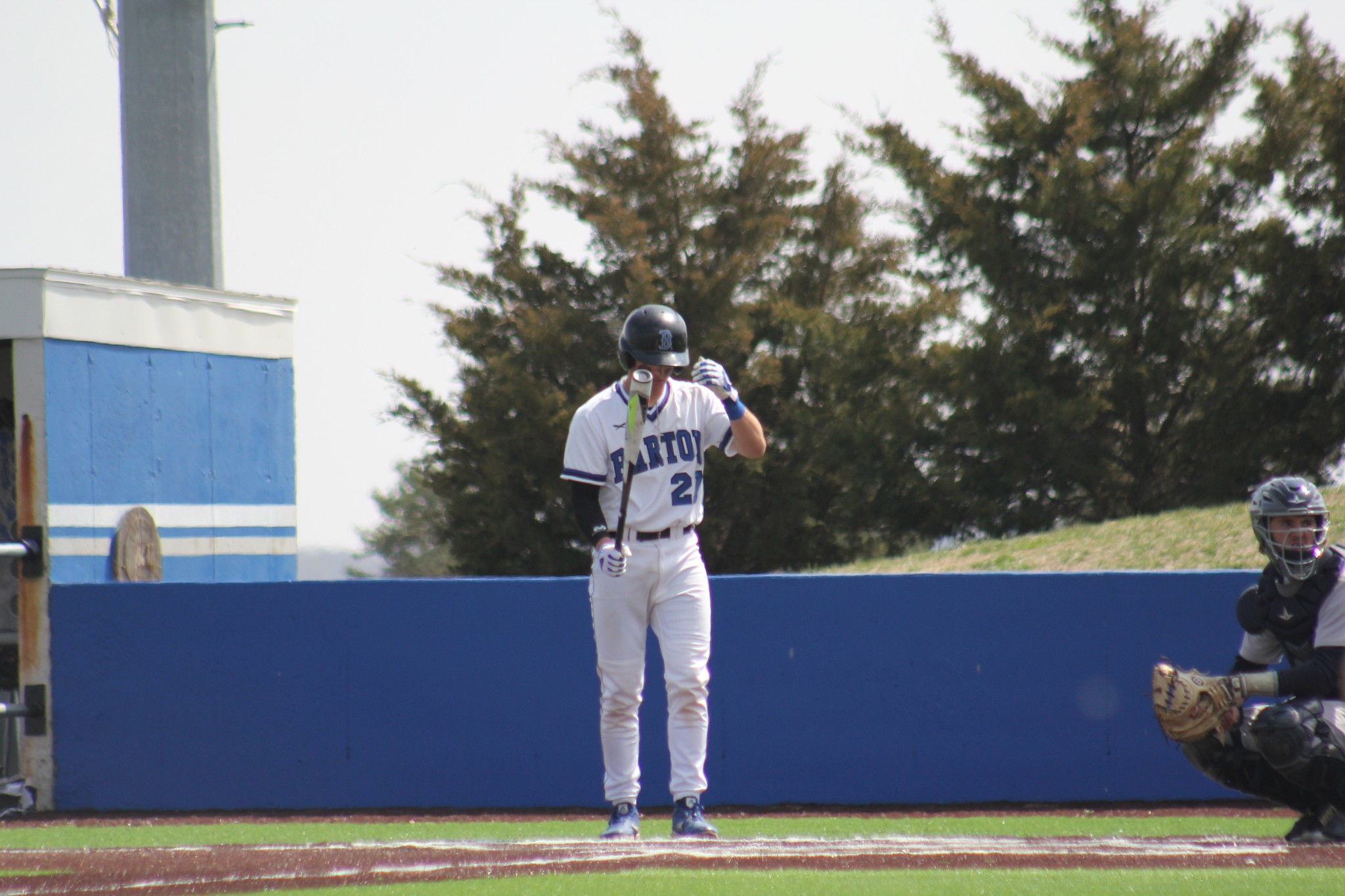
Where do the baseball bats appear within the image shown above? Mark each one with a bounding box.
[615,369,653,551]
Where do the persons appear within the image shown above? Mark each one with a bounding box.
[560,303,768,840]
[1151,476,1345,842]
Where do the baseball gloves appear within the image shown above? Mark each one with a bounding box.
[1152,662,1246,743]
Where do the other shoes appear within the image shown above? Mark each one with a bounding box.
[1286,805,1345,842]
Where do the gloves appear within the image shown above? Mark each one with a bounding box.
[598,542,632,577]
[691,356,738,403]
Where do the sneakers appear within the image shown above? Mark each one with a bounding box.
[599,802,640,839]
[670,796,718,839]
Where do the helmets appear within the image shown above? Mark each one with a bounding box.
[618,304,689,375]
[1250,476,1330,573]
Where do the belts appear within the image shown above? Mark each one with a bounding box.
[629,525,691,540]
